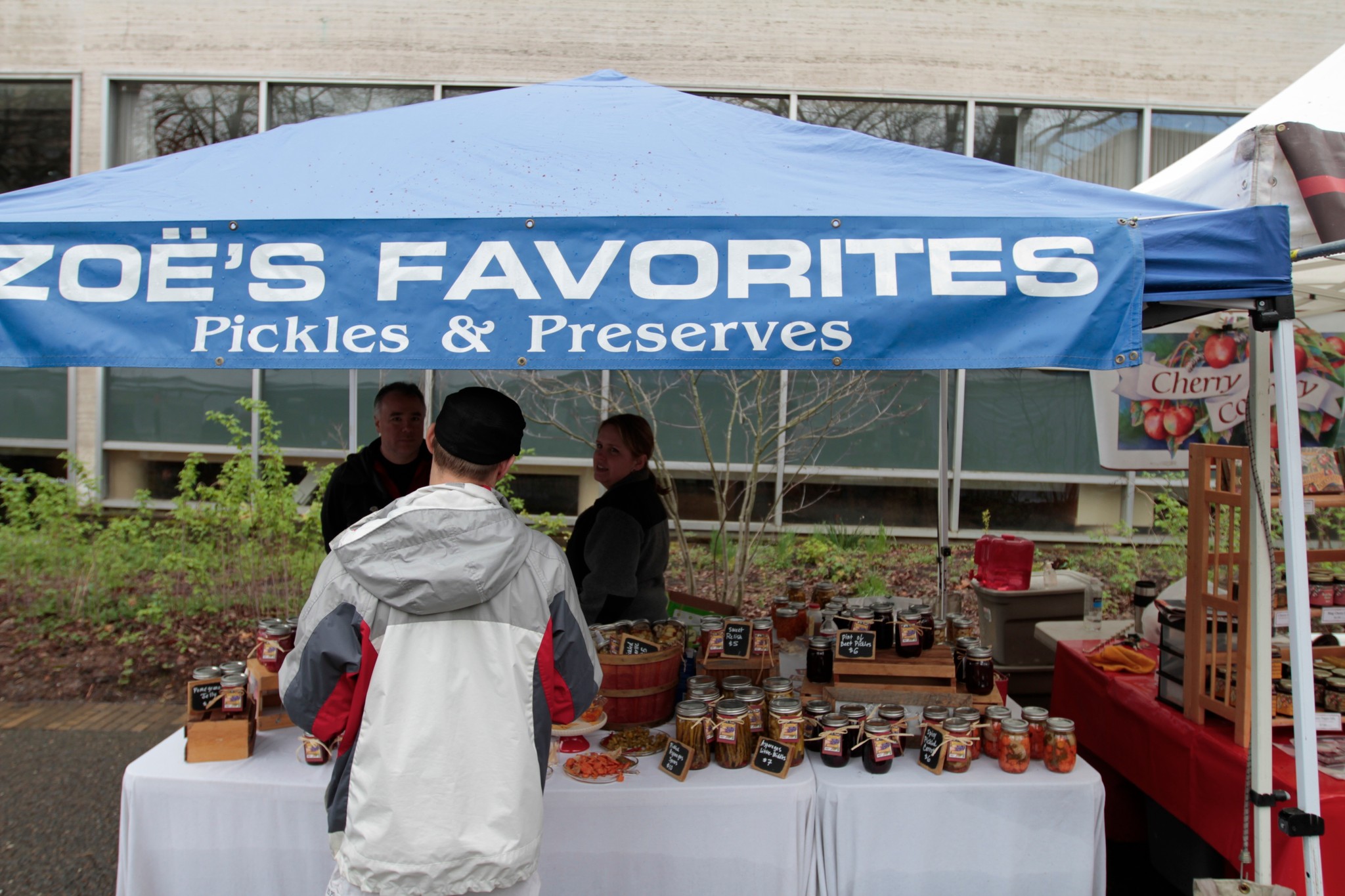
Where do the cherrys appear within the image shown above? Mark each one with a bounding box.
[1140,330,1344,447]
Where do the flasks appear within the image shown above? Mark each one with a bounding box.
[1132,579,1158,633]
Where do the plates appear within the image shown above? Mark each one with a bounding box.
[550,713,670,788]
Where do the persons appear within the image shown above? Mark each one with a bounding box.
[275,386,605,895]
[319,381,433,556]
[562,413,672,628]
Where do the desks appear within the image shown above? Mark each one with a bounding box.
[1035,617,1342,895]
[115,689,1107,896]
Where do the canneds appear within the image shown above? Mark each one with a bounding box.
[189,580,1076,782]
[1229,567,1345,719]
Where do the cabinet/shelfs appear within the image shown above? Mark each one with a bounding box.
[1181,443,1345,749]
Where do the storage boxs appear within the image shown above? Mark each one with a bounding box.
[993,666,1055,710]
[971,568,1105,665]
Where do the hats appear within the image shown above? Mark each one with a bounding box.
[435,386,526,466]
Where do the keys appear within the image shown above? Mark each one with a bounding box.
[1112,632,1154,652]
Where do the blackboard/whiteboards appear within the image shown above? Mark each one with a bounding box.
[188,677,222,715]
[918,723,951,776]
[658,738,695,783]
[834,630,876,660]
[751,734,796,779]
[721,620,754,659]
[619,633,659,657]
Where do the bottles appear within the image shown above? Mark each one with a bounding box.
[1084,578,1104,632]
[819,615,838,648]
[969,530,1036,592]
[805,602,822,642]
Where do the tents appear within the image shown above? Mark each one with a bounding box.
[0,67,1329,896]
[1086,39,1345,896]
